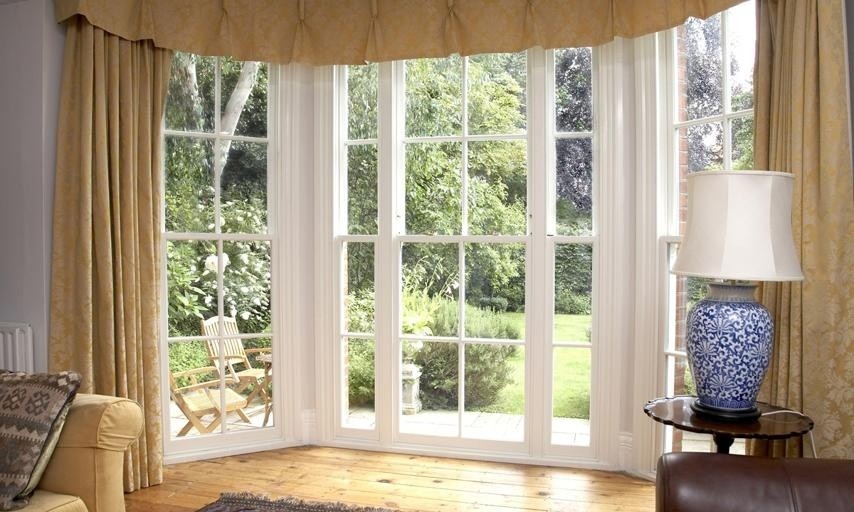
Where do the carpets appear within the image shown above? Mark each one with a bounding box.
[196,491,402,512]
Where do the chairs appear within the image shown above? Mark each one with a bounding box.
[655,452,854,512]
[169,366,251,437]
[199,315,271,425]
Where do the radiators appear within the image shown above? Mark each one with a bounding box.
[0,322,34,374]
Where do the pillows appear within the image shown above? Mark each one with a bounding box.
[0,369,82,512]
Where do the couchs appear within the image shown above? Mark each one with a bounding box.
[0,391,145,512]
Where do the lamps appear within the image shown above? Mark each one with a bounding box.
[667,171,803,424]
